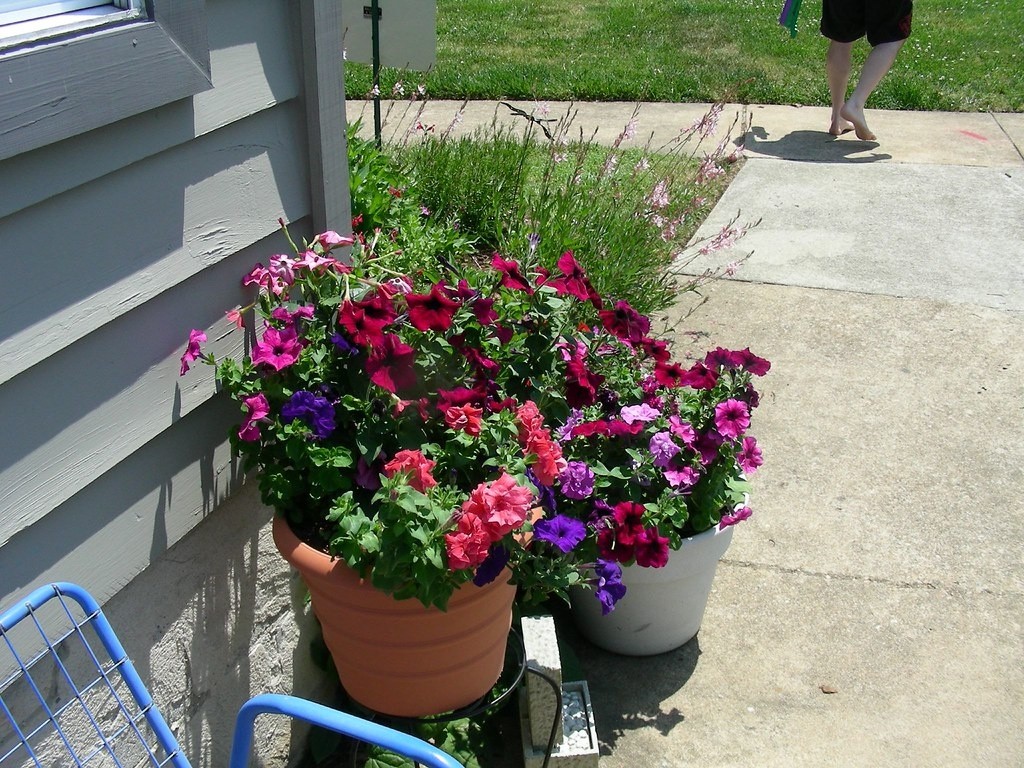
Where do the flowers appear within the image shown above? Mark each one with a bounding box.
[190,78,791,608]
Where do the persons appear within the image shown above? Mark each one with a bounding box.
[820,0,914,141]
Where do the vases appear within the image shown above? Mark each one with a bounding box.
[267,501,549,717]
[562,465,751,660]
